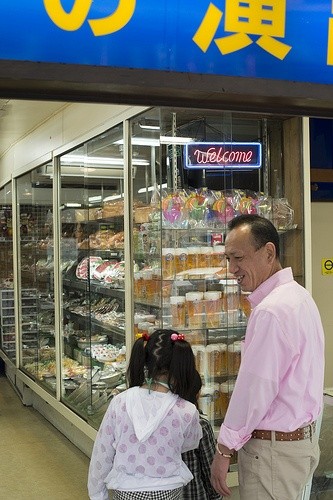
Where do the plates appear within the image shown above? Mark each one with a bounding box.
[76,255,124,284]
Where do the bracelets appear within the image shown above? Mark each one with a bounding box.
[215,443,234,461]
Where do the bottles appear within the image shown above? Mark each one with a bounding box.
[134,245,251,421]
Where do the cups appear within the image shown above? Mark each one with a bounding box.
[271,197,295,231]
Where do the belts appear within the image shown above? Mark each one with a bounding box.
[252,421,317,442]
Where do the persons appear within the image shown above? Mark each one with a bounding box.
[85,329,204,500]
[207,215,327,498]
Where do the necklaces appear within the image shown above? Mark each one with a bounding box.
[139,366,175,396]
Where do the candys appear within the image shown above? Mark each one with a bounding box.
[161,190,259,226]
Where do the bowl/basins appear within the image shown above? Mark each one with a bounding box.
[99,372,122,384]
[67,382,109,418]
[77,339,107,350]
